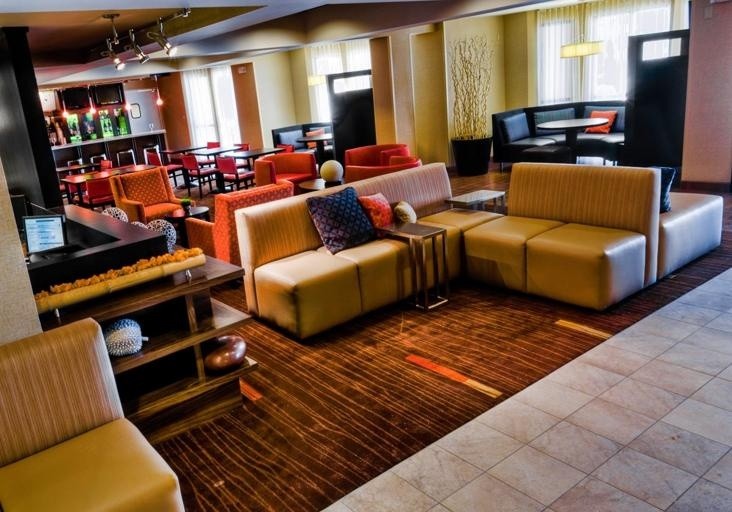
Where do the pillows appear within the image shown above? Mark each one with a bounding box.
[532,106,577,134]
[583,104,626,130]
[650,165,677,212]
[500,111,531,144]
[586,109,618,134]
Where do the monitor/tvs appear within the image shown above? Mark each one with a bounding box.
[91,84,124,106]
[11,194,28,229]
[20,214,68,257]
[61,86,91,110]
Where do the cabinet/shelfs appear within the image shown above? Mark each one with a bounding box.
[39,246,261,441]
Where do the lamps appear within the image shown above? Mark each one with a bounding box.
[559,3,603,59]
[97,9,195,72]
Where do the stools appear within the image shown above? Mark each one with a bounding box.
[522,144,576,162]
[660,189,725,275]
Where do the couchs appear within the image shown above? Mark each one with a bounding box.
[108,167,195,226]
[233,158,506,343]
[462,161,663,312]
[185,181,293,262]
[490,102,629,164]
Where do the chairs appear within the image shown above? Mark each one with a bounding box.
[53,150,157,214]
[0,313,187,512]
[148,126,423,199]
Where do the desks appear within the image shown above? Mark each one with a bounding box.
[534,118,609,162]
[383,216,452,310]
[449,134,494,175]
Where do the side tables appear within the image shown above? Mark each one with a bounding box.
[162,204,209,244]
[445,187,505,214]
